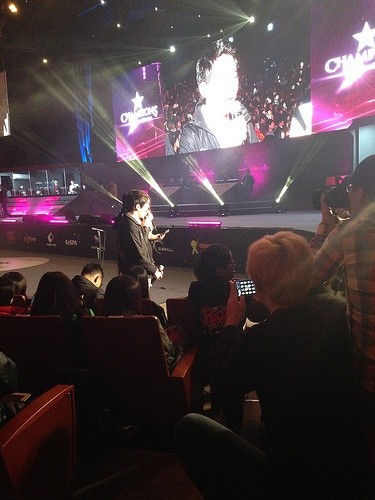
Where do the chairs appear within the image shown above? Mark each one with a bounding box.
[0,304,203,500]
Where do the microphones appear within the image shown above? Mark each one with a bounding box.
[147,212,157,231]
[230,99,247,146]
[150,266,164,287]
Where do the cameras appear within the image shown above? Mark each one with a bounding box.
[312,179,353,211]
[234,279,257,297]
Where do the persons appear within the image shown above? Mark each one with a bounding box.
[187,244,246,434]
[0,272,194,426]
[167,39,307,155]
[117,190,163,277]
[218,230,369,500]
[309,155,375,475]
[0,179,79,217]
[241,168,256,200]
